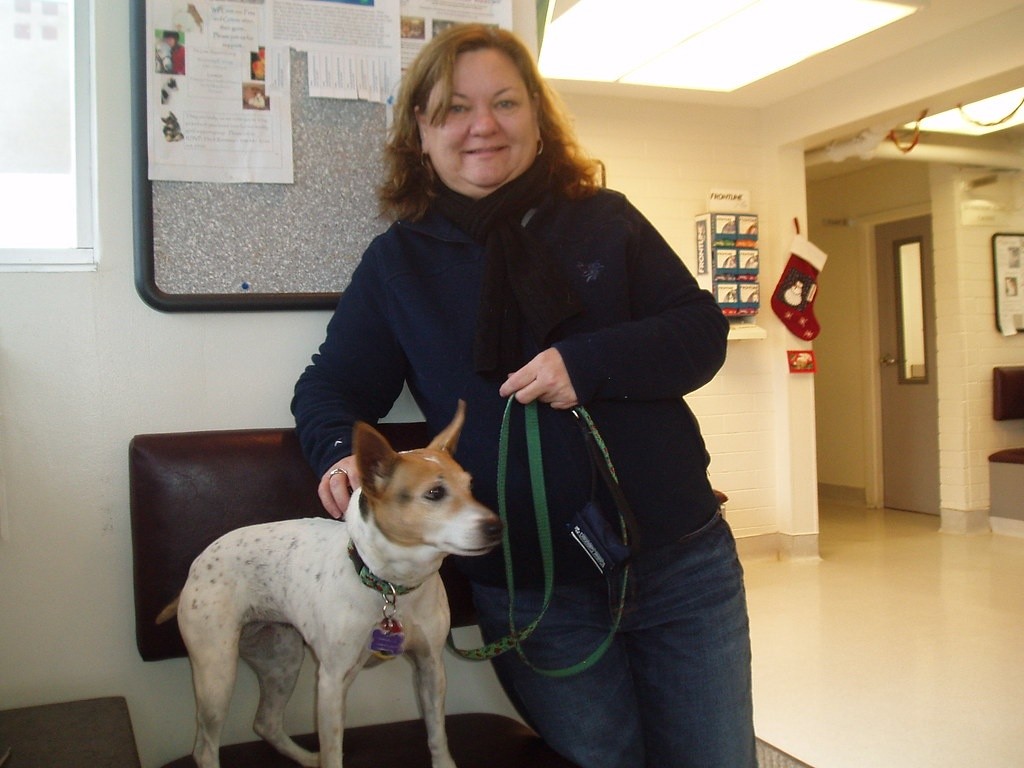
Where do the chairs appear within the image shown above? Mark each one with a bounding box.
[128,420,574,768]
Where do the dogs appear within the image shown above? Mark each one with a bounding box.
[160,402,500,762]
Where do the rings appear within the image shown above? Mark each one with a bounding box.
[328,467,347,480]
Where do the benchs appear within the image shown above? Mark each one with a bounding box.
[989,365,1024,521]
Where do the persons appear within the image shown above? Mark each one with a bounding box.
[163,30,186,75]
[290,22,759,768]
